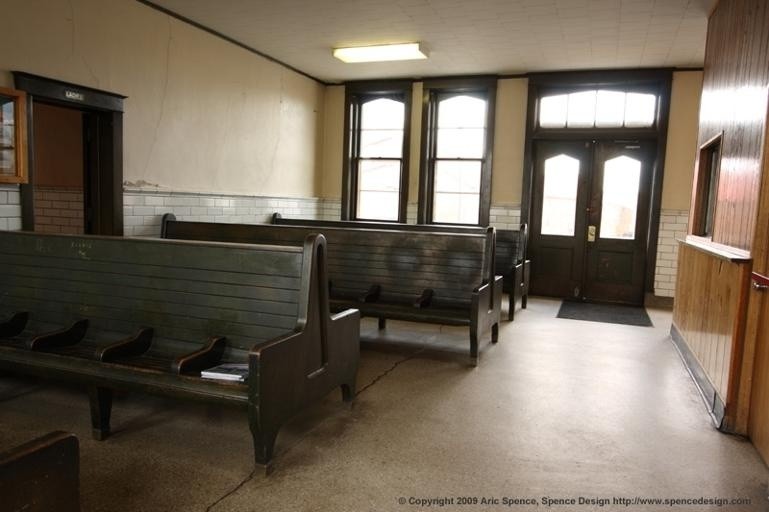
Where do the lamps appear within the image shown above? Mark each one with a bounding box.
[332,40,428,64]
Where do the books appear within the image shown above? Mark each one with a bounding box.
[200,363,249,382]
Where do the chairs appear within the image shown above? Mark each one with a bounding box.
[0,213,534,511]
